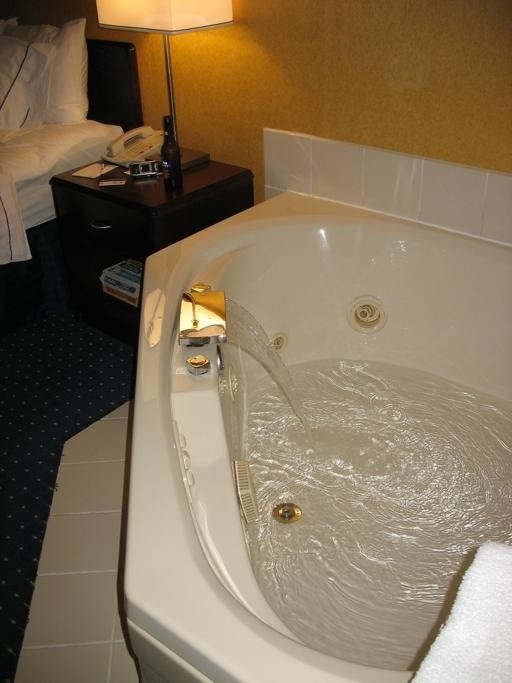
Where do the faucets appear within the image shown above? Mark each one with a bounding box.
[180,291,228,348]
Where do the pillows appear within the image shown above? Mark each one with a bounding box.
[0,18,89,127]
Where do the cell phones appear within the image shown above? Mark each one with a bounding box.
[129,160,164,177]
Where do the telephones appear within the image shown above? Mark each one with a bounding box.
[102,126,165,169]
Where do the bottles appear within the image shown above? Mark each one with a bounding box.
[160,114,184,192]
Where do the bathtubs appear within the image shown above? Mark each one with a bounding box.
[123,190,512,682]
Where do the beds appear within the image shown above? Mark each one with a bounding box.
[0,38,147,234]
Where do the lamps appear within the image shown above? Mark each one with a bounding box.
[94,0,235,177]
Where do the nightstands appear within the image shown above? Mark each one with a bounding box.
[49,150,254,346]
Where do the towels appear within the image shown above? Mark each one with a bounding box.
[408,538,510,679]
